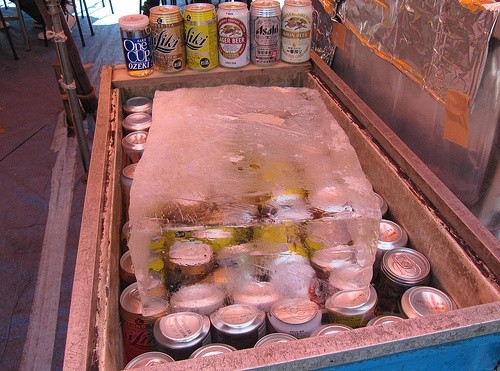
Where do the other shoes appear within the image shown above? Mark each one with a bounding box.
[0,21,10,28]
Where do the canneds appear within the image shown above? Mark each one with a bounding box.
[281,0,312,63]
[217,1,251,69]
[149,5,186,72]
[249,0,282,66]
[182,3,219,71]
[120,97,458,371]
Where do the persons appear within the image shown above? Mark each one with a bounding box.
[9,0,77,42]
